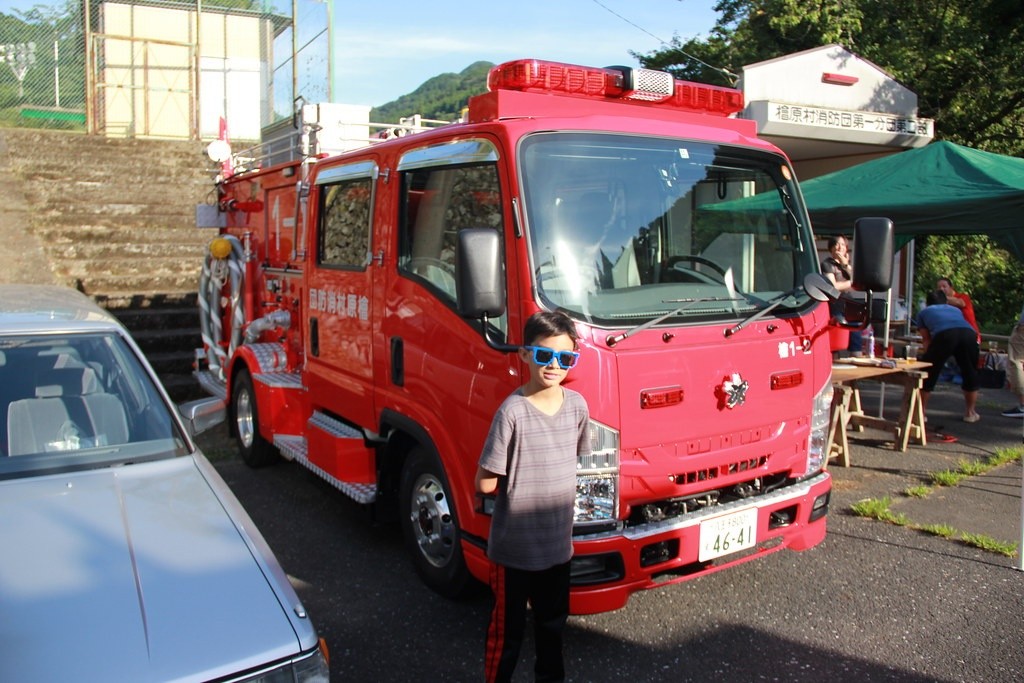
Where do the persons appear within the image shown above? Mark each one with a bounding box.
[473,311,591,683]
[821,234,861,361]
[1000,308,1024,419]
[932,278,981,385]
[916,289,981,424]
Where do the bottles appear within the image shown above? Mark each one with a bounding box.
[867,331,875,357]
[919,299,926,311]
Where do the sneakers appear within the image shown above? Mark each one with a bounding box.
[1001,406,1024,417]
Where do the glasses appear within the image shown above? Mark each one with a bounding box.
[524,345,580,368]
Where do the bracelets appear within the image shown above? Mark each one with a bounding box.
[843,265,848,269]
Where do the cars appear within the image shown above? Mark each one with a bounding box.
[0,282,330,683]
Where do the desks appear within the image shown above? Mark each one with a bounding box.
[825,359,933,470]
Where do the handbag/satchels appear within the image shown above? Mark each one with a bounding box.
[976,349,1006,389]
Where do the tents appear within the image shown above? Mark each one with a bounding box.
[695,138,1024,420]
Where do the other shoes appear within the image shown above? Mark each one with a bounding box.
[964,412,980,423]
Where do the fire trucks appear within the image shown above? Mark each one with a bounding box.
[193,59,898,615]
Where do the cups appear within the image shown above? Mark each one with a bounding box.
[905,345,919,364]
[988,341,998,354]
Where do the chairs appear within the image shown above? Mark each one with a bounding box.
[8,350,128,456]
